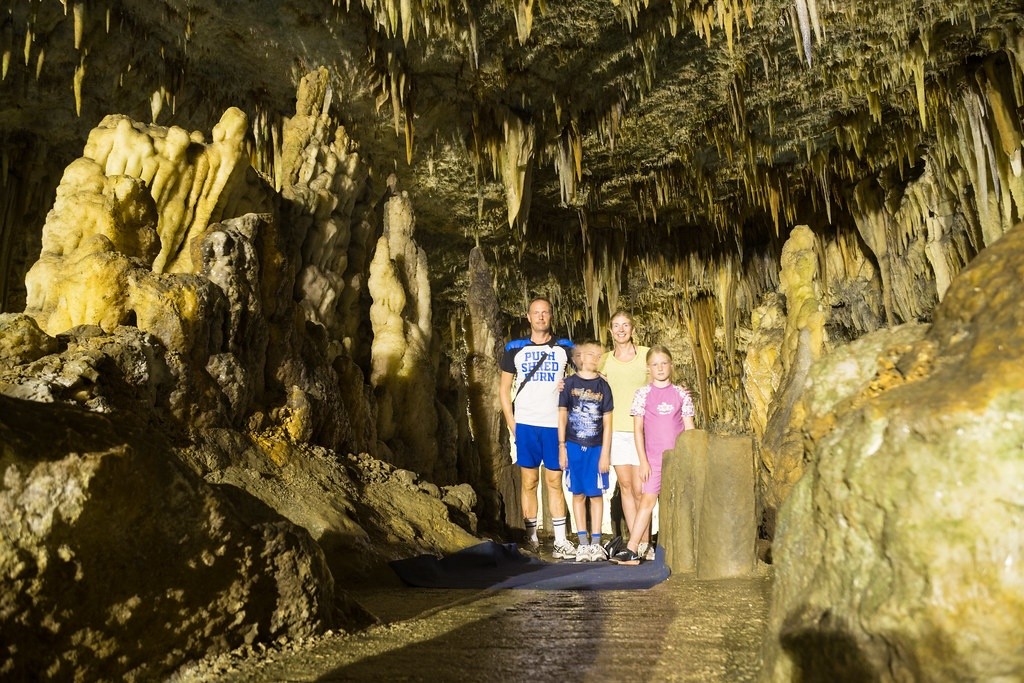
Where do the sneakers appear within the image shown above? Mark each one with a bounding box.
[524,535,541,558]
[609,548,641,566]
[553,539,577,559]
[576,543,592,563]
[638,542,655,560]
[590,543,609,562]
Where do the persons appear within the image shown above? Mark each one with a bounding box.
[501,298,581,560]
[559,310,692,560]
[612,344,697,565]
[556,333,614,564]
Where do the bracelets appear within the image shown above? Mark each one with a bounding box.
[559,441,566,445]
